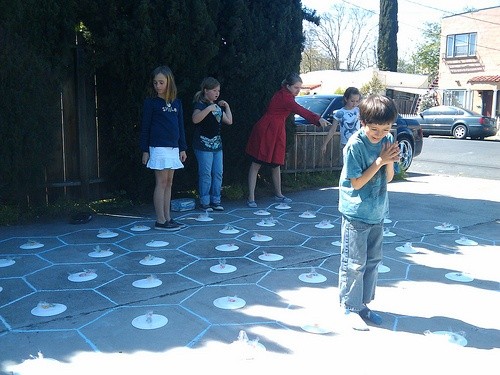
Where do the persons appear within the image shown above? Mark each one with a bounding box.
[140,65,187,231]
[320,87,361,202]
[239,72,332,208]
[337,94,402,331]
[191,77,233,212]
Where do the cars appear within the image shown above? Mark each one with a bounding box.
[418,106,498,138]
[293,94,429,172]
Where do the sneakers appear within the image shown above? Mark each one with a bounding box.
[155,218,185,231]
[199,204,213,212]
[211,202,224,210]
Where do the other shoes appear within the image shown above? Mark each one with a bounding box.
[274,195,292,203]
[247,198,257,207]
